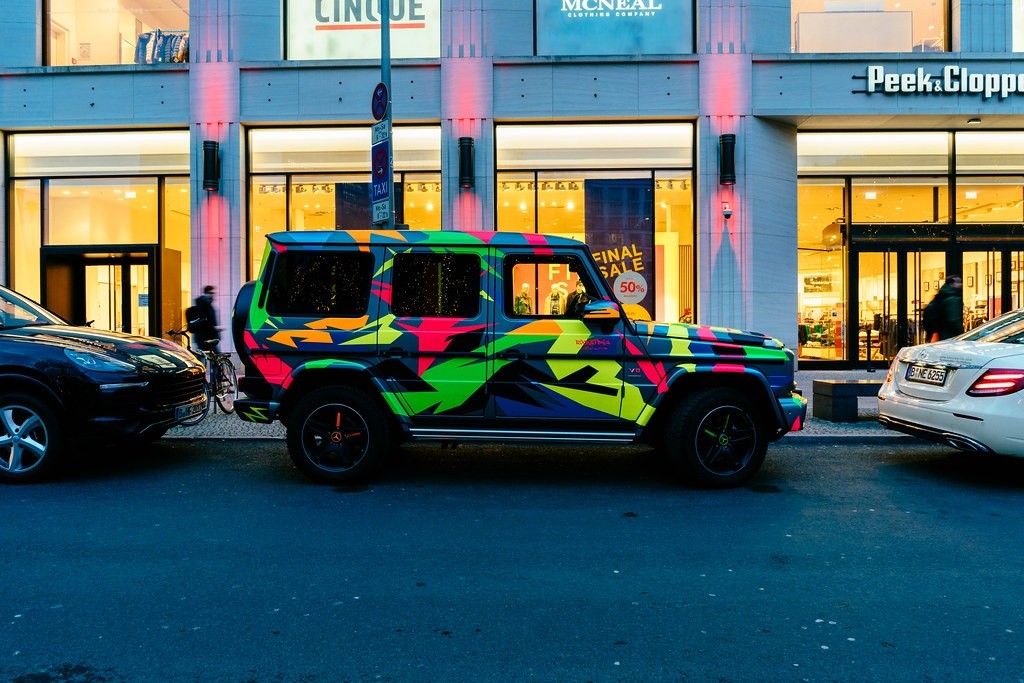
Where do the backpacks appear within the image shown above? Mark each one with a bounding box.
[186,306,209,333]
[923,293,953,334]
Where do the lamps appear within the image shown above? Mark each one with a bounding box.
[527,182,535,190]
[406,184,415,193]
[967,118,981,124]
[458,137,475,188]
[719,134,736,186]
[203,140,220,191]
[258,184,334,194]
[515,182,525,191]
[655,180,662,190]
[665,181,674,190]
[420,184,428,192]
[555,182,565,191]
[678,180,688,190]
[501,182,510,192]
[542,182,553,191]
[435,184,440,192]
[568,182,579,191]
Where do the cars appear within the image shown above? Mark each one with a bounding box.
[0,281,209,487]
[876,308,1023,457]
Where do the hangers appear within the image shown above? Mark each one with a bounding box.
[145,29,190,37]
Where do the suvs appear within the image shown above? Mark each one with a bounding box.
[232,231,807,489]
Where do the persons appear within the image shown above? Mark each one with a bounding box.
[567,281,587,307]
[922,274,964,342]
[514,283,532,314]
[189,285,229,400]
[545,284,566,316]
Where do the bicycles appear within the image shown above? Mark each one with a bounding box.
[163,316,238,425]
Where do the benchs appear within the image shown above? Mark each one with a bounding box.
[812,379,884,422]
